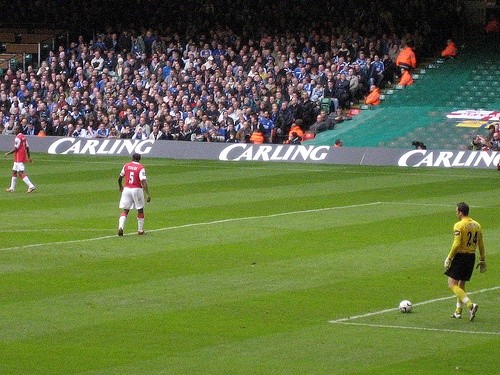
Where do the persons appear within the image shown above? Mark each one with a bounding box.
[4,125,35,193]
[441,38,458,58]
[334,139,343,148]
[443,202,487,321]
[0,0,433,145]
[467,123,500,152]
[117,153,151,236]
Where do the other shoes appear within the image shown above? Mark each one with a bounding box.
[26,186,35,193]
[469,303,478,321]
[6,187,16,192]
[118,227,123,236]
[138,230,144,235]
[450,312,463,318]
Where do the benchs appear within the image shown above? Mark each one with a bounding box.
[0,23,71,71]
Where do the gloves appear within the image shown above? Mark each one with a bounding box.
[444,257,453,271]
[476,260,487,273]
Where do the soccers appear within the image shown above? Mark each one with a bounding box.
[399,300,412,312]
